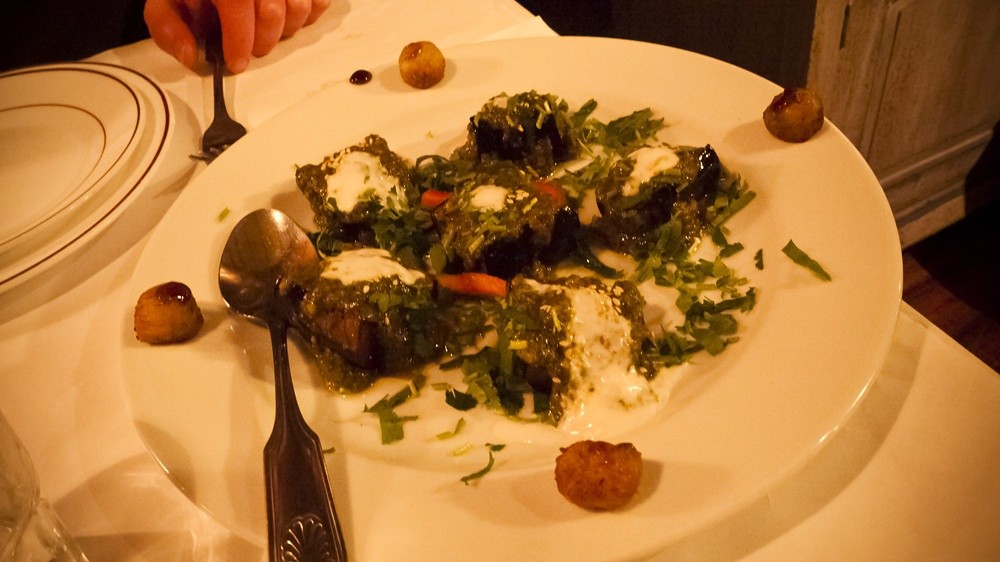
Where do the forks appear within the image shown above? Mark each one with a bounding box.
[196,0,245,167]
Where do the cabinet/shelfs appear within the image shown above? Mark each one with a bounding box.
[615,2,999,260]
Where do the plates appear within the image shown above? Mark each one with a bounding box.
[120,32,910,558]
[0,58,169,292]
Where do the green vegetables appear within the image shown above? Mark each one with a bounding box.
[289,90,759,447]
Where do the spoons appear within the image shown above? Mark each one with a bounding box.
[216,203,344,562]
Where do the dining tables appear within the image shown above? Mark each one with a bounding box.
[0,2,1000,562]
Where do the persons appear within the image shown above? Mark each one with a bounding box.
[0,0,338,78]
